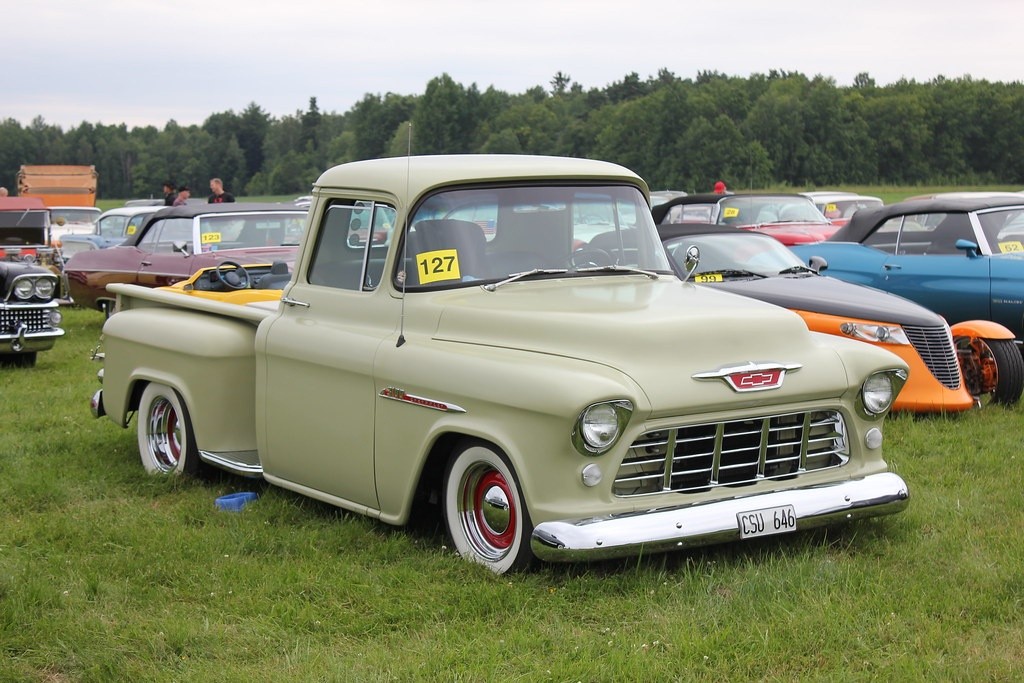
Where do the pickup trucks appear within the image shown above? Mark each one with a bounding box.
[91,151,909,578]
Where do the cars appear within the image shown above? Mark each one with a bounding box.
[59,204,309,308]
[0,188,312,246]
[649,189,1024,239]
[0,255,65,369]
[787,191,1024,346]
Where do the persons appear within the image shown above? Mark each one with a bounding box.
[162,182,177,205]
[172,185,191,206]
[208,178,235,202]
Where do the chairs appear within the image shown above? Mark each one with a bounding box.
[259,261,292,290]
[324,210,571,290]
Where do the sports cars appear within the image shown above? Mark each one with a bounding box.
[592,222,1023,415]
